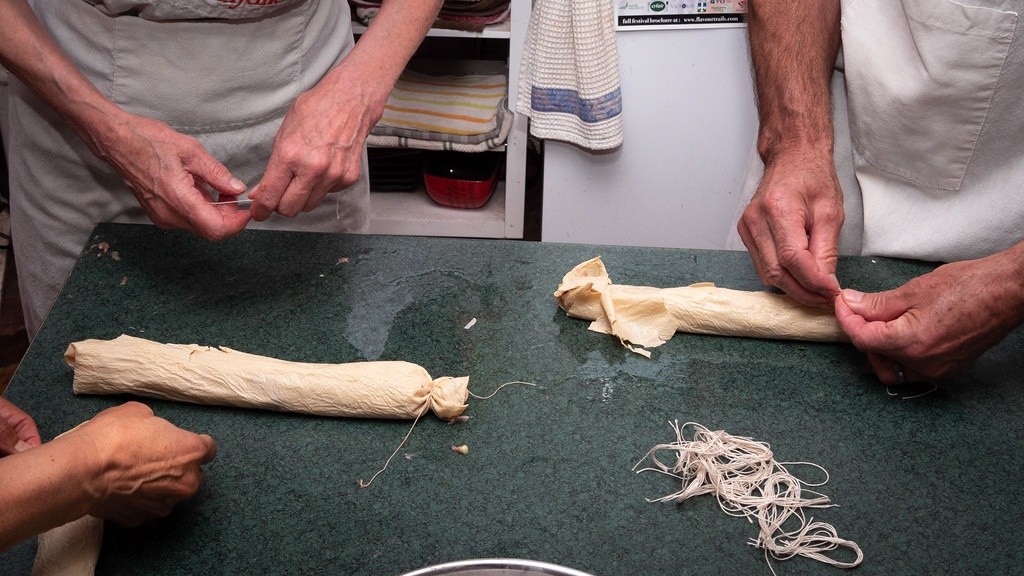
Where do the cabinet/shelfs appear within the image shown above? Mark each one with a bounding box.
[351,0,532,239]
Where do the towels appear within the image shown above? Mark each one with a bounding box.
[366,67,515,153]
[514,0,625,152]
[346,0,511,33]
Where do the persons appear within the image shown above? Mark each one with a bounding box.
[0,0,448,340]
[0,397,216,553]
[736,0,1024,392]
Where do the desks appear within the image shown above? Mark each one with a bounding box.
[0,221,1024,576]
